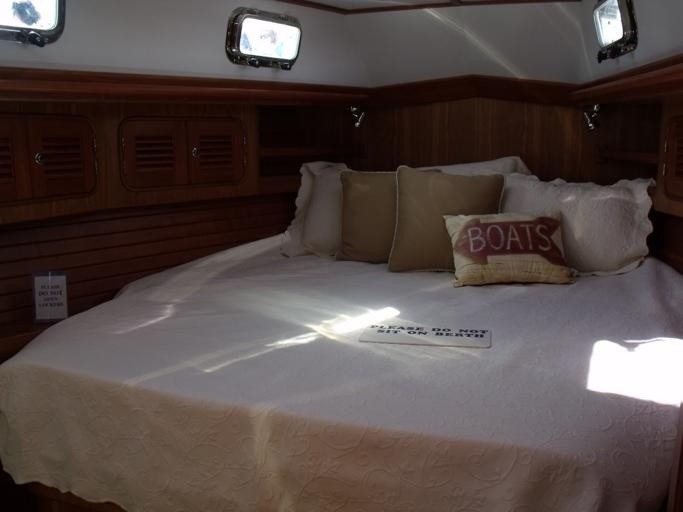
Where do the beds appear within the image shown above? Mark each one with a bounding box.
[0,227,683,512]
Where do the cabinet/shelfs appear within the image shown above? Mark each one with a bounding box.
[567,53,683,218]
